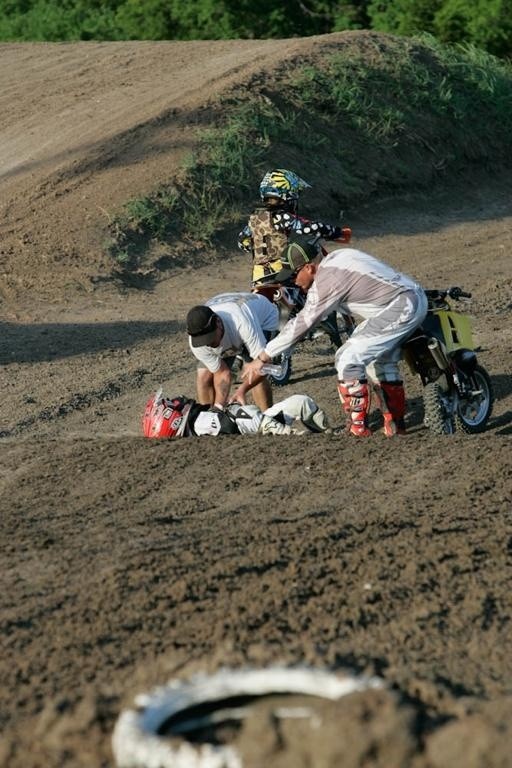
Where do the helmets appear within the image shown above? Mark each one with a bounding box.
[259,168,313,203]
[142,387,186,439]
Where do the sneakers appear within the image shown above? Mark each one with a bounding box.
[325,424,372,437]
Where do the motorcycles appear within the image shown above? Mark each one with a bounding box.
[403,287,494,436]
[251,228,356,387]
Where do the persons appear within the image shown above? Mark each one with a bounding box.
[186,291,279,411]
[140,385,341,439]
[239,239,429,437]
[237,171,351,289]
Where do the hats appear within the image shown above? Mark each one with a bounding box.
[185,305,219,347]
[274,240,320,282]
